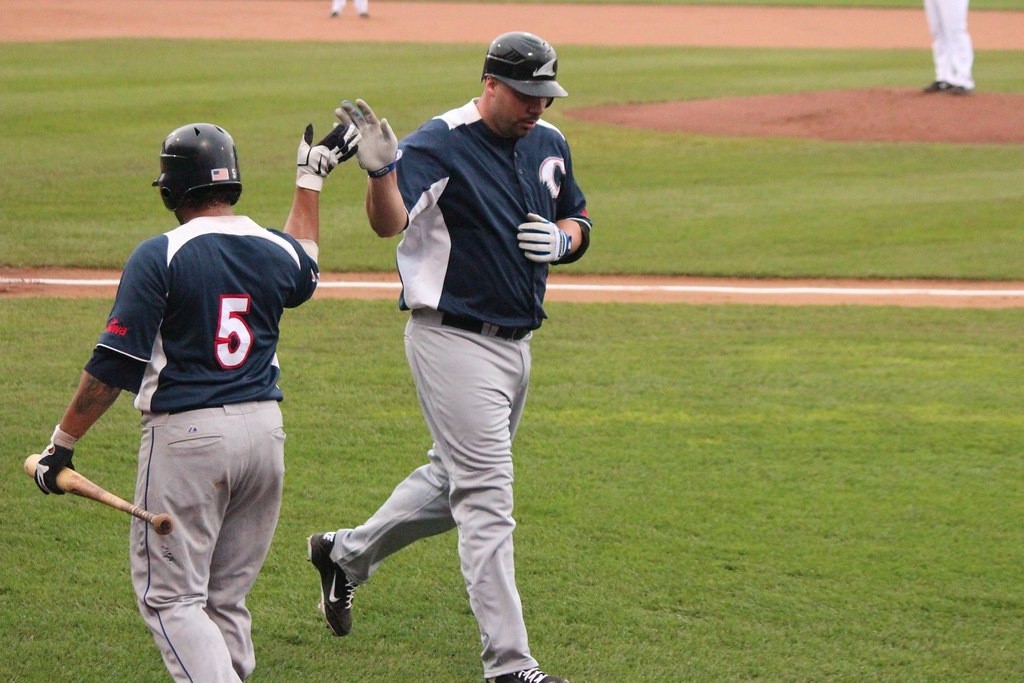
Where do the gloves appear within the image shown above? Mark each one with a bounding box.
[295,123,361,195]
[334,100,403,176]
[518,212,571,264]
[32,423,75,497]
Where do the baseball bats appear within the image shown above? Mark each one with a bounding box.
[23,453,174,536]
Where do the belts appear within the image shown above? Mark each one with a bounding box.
[442,309,532,342]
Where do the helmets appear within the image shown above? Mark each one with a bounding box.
[151,122,245,195]
[481,33,568,108]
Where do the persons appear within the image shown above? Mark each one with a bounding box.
[307,31,592,683]
[923,0,975,96]
[35,122,362,683]
[331,1,370,19]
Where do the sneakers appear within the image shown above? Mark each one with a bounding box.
[330,12,370,19]
[922,80,969,96]
[304,531,359,637]
[484,667,569,683]
